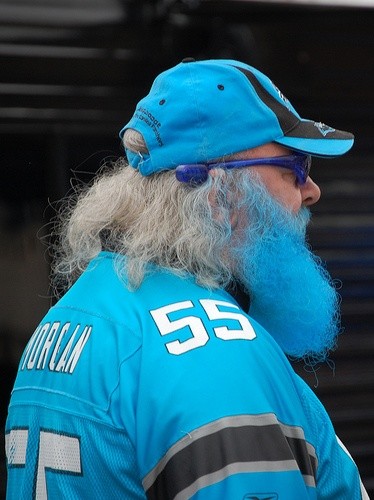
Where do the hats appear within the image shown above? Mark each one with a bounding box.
[120,58,354,177]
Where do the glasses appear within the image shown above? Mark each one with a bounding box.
[176,153,311,185]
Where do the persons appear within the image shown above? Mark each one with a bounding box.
[6,59,374,500]
